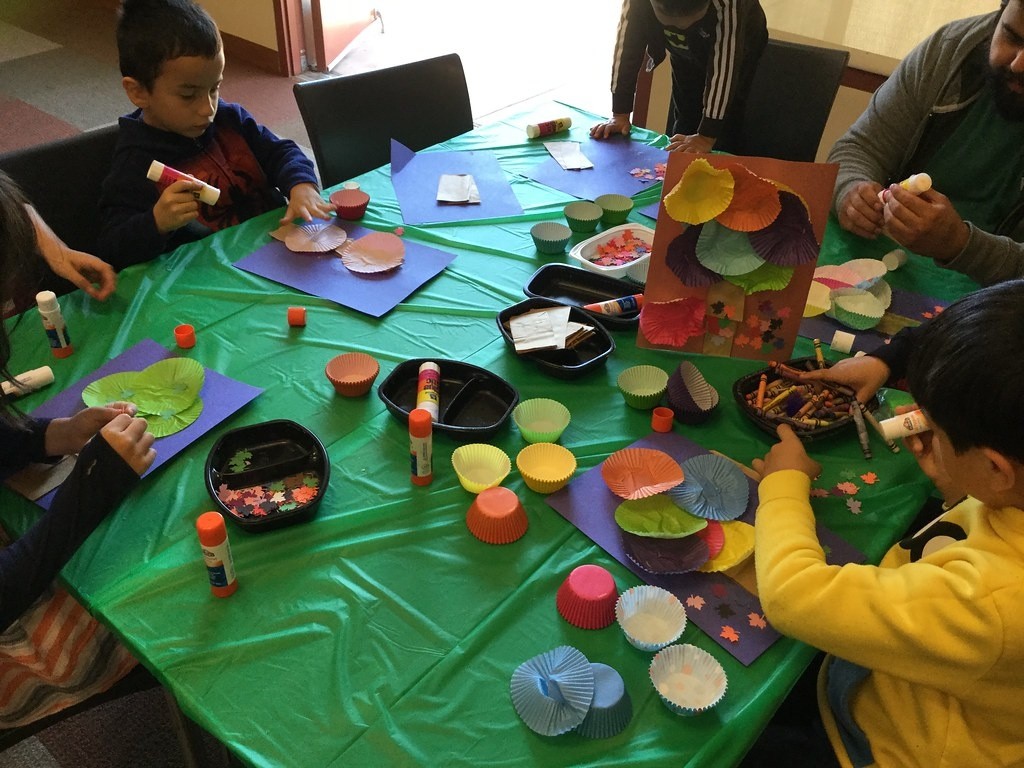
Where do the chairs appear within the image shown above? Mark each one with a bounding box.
[664,38,850,163]
[0,123,124,303]
[292,53,475,190]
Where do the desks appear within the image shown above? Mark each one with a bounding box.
[1,101,989,767]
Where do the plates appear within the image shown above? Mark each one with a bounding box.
[568,221,655,280]
[732,354,883,443]
[377,358,519,443]
[523,261,647,328]
[495,296,618,378]
[204,419,331,534]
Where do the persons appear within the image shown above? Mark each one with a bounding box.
[96,0,337,271]
[825,0,1024,288]
[0,172,158,729]
[752,280,1024,768]
[590,0,769,158]
